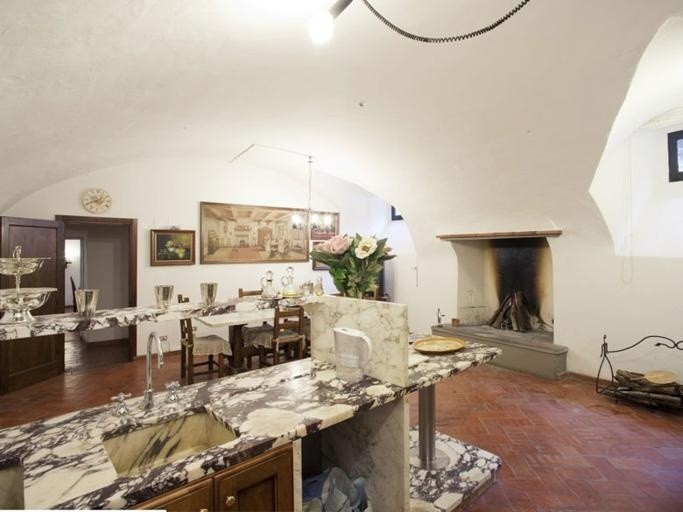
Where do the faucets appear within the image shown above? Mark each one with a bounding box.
[138,332,164,409]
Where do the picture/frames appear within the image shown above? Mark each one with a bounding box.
[391,204,403,221]
[151,229,195,266]
[311,212,339,240]
[313,243,330,270]
[200,202,308,264]
[667,130,683,182]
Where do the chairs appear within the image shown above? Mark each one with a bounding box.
[242,306,304,368]
[178,295,224,385]
[238,288,263,368]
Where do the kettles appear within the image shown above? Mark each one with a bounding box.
[332,327,372,382]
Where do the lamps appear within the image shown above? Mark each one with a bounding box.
[307,0,352,48]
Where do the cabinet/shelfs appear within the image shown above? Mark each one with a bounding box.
[125,443,294,512]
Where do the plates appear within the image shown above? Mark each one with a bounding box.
[412,337,465,354]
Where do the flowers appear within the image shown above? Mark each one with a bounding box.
[308,233,397,299]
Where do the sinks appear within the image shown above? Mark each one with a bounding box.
[100,405,240,479]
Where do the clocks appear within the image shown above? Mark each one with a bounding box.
[82,188,112,214]
